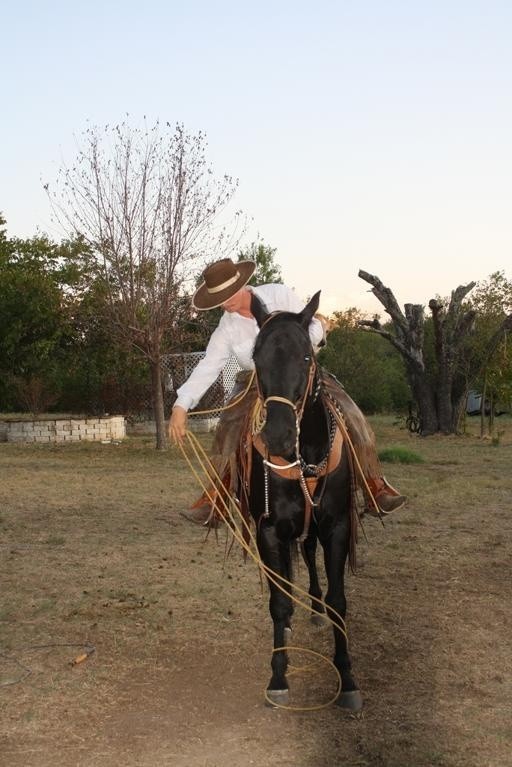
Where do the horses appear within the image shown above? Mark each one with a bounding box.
[244,289,365,711]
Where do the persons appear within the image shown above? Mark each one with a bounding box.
[163,256,407,530]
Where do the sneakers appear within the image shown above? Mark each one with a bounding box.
[180,503,219,528]
[370,493,407,517]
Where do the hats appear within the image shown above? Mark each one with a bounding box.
[192,258,256,311]
